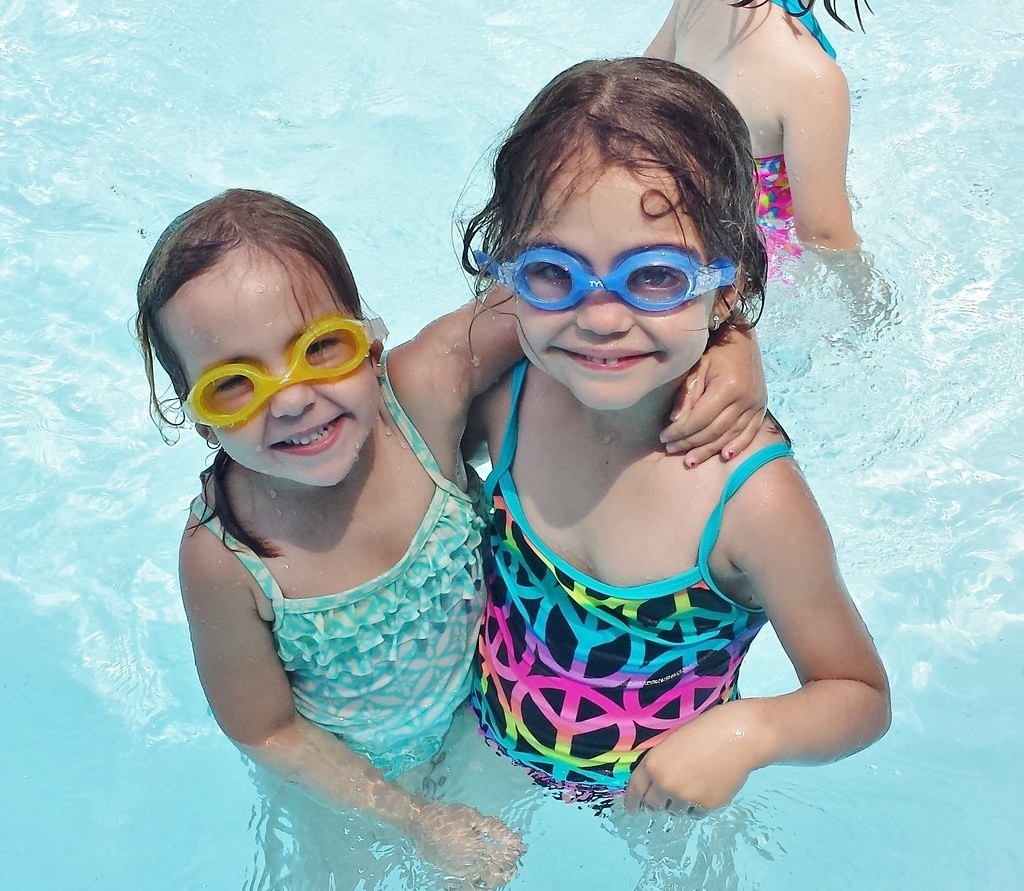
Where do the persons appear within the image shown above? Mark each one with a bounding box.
[461,54,892,814]
[137,189,768,744]
[645,0,875,251]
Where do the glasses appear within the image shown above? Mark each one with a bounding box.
[186,313,372,431]
[510,245,705,312]
